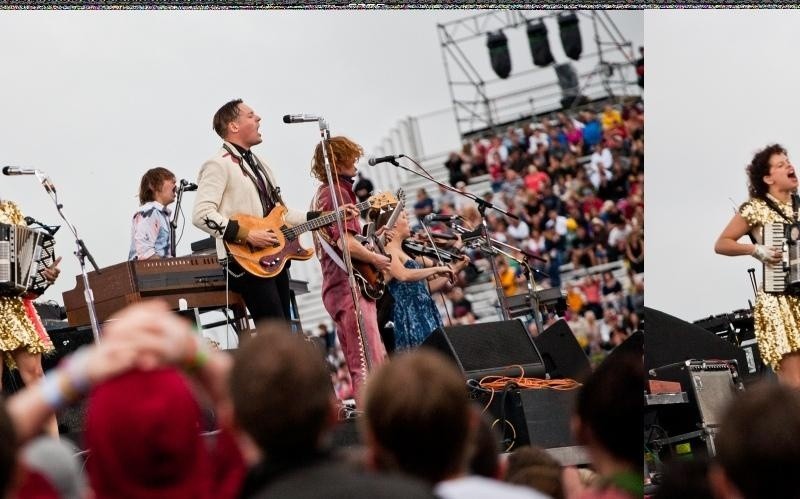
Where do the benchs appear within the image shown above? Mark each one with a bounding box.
[395,152,644,327]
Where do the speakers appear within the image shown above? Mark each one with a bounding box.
[534,319,590,378]
[647,359,747,438]
[473,387,534,455]
[419,319,545,380]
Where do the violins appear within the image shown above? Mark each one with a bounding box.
[404,239,483,273]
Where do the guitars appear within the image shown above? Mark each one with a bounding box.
[344,186,406,302]
[222,194,398,279]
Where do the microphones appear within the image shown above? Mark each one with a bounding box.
[2,163,41,178]
[283,112,324,122]
[172,180,197,190]
[368,154,401,169]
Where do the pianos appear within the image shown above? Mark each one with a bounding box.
[61,254,308,329]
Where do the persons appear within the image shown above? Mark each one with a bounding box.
[0,94,643,499]
[648,460,720,499]
[633,46,644,92]
[714,144,799,388]
[706,379,799,499]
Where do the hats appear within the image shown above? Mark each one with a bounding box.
[84,365,216,499]
